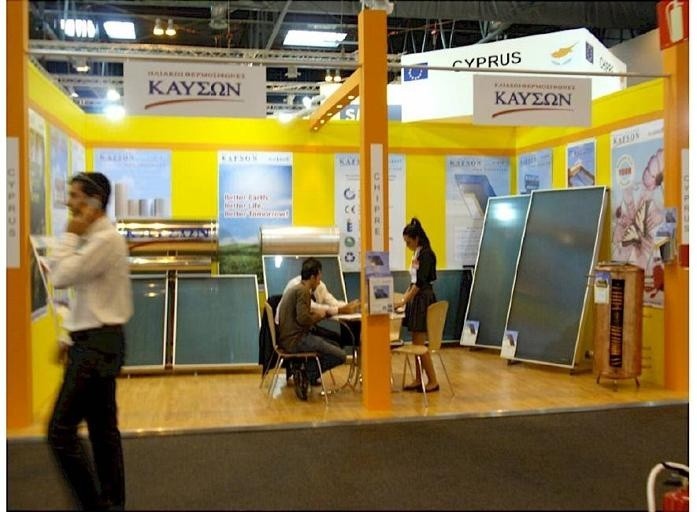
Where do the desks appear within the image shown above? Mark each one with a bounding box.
[319,309,405,396]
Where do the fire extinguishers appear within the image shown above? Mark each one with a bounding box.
[660,461,689,512]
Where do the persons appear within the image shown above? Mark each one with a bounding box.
[43,172,136,511]
[275,255,362,389]
[392,218,439,394]
[276,262,348,401]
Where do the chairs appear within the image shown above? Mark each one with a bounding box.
[388,300,455,406]
[257,291,355,405]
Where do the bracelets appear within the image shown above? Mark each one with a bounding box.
[401,297,405,304]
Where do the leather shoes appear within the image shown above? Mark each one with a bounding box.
[404,384,439,392]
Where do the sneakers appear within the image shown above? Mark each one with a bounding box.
[294,370,308,400]
